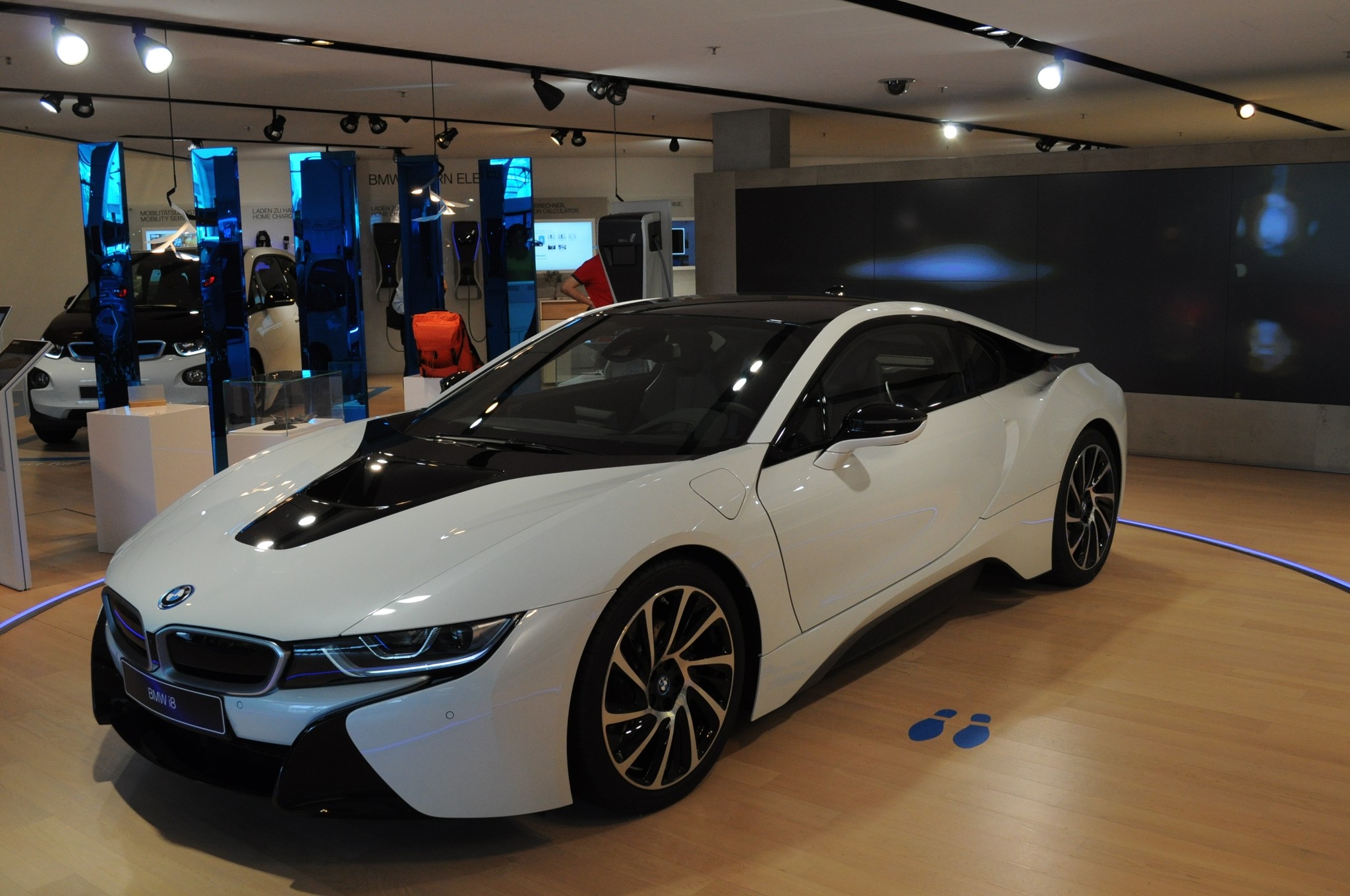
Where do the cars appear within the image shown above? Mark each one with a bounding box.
[21,243,302,445]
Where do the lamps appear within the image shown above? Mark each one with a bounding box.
[340,113,360,134]
[550,128,569,146]
[571,130,586,147]
[367,115,388,134]
[669,138,680,152]
[49,14,90,66]
[586,79,629,105]
[531,73,565,111]
[1234,102,1256,119]
[39,92,64,114]
[72,95,95,118]
[436,120,458,149]
[1037,54,1066,90]
[264,109,286,142]
[131,26,174,75]
[1036,137,1092,152]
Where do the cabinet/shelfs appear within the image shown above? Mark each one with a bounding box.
[537,298,589,383]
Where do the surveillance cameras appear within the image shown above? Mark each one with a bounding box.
[879,78,915,95]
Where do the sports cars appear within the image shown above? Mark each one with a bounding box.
[89,294,1130,827]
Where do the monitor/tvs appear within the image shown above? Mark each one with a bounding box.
[0,339,53,395]
[672,228,685,255]
[534,218,596,272]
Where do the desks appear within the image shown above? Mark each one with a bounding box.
[86,403,213,552]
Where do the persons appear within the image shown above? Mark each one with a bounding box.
[560,253,614,311]
[392,277,448,377]
